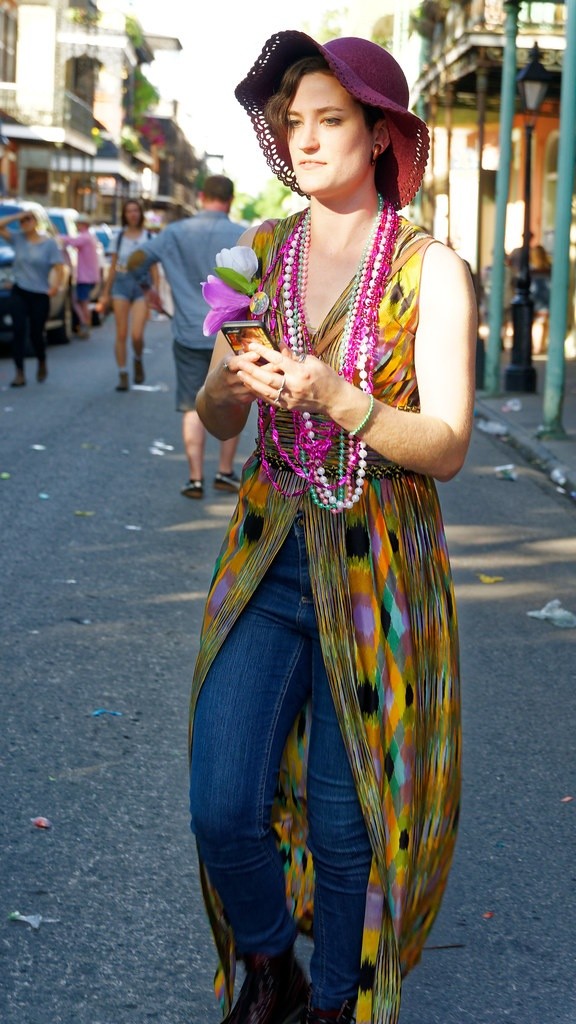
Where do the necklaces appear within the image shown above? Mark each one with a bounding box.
[251,193,399,514]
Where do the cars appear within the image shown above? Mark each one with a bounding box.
[1,198,166,344]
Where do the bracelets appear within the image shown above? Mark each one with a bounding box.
[349,394,375,436]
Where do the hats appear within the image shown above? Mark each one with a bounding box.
[234,30,429,211]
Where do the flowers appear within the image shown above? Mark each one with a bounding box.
[200,246,261,338]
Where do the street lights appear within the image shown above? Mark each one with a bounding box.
[503,40,551,392]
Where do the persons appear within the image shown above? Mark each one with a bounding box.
[96,199,160,391]
[0,202,65,388]
[484,232,552,355]
[187,30,478,1024]
[61,213,101,339]
[126,175,248,499]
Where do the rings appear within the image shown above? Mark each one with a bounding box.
[298,354,306,363]
[276,378,285,390]
[223,361,229,371]
[273,390,282,404]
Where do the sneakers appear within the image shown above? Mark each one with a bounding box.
[181,479,203,500]
[213,470,240,492]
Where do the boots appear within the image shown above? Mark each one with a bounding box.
[116,372,129,392]
[134,358,144,384]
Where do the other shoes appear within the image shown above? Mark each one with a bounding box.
[10,371,26,386]
[220,953,358,1024]
[37,360,46,382]
